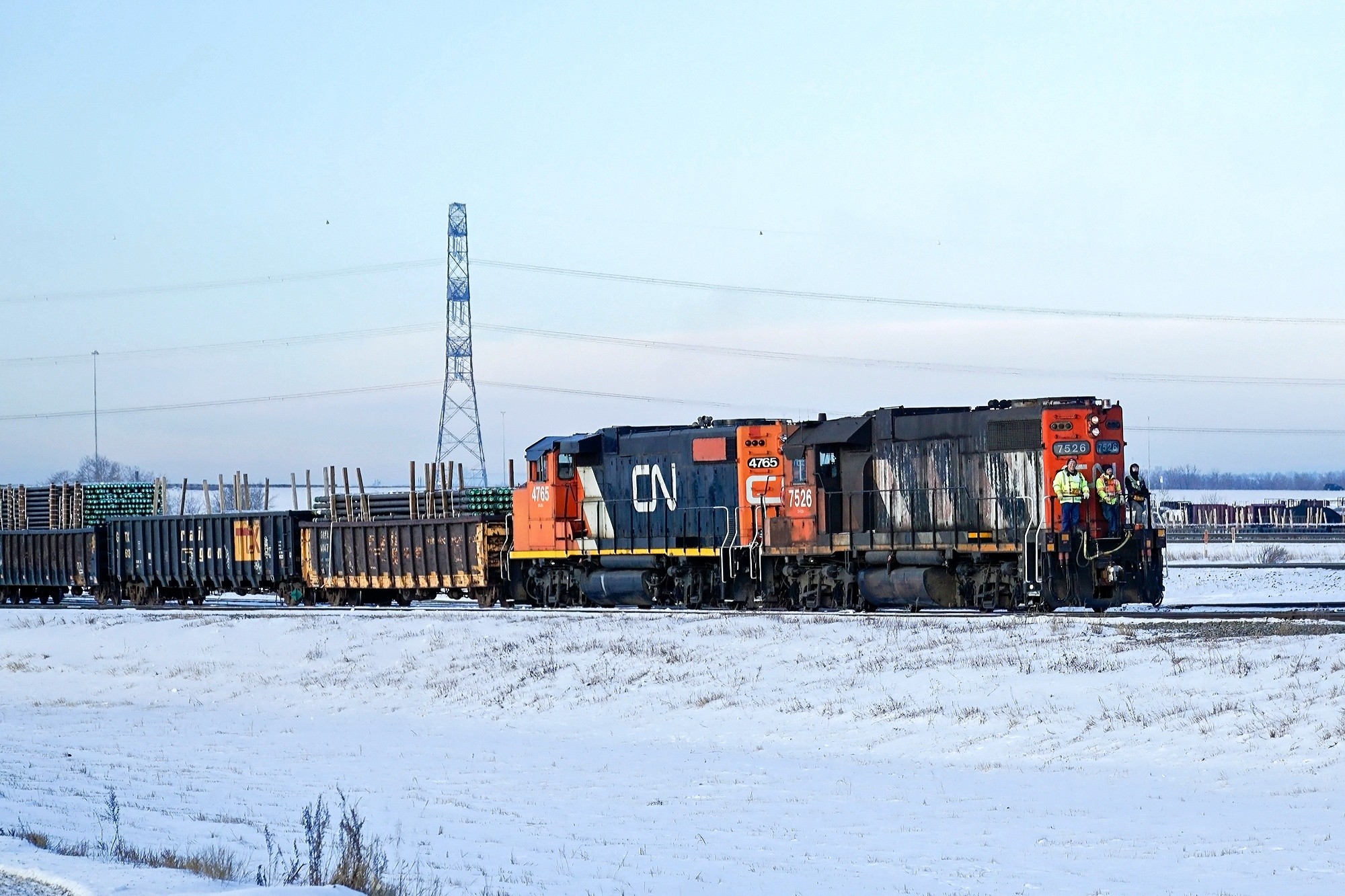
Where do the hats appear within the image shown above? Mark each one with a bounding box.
[1067,458,1077,467]
[1104,465,1113,476]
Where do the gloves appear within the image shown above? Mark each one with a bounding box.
[1083,492,1089,499]
[1104,496,1110,502]
[1057,493,1062,500]
[1120,495,1125,505]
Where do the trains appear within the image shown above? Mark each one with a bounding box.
[1,394,1171,621]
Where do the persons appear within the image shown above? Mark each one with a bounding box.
[1096,464,1125,538]
[1052,458,1090,533]
[1125,463,1148,529]
